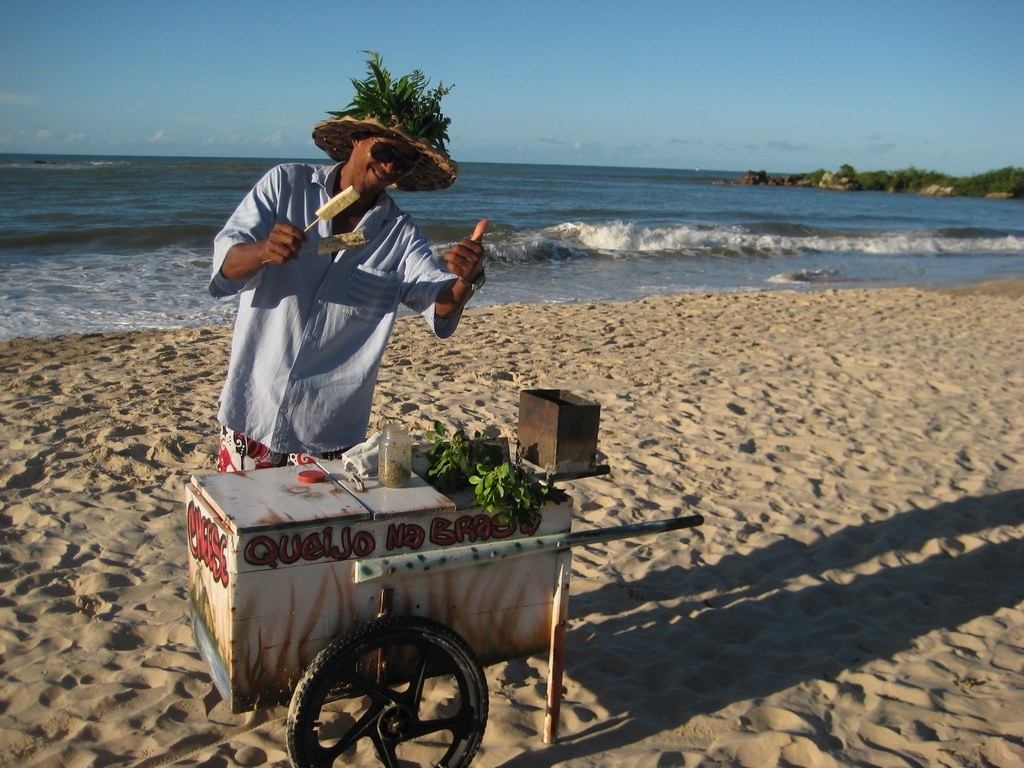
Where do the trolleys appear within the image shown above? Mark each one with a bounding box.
[184,389,705,768]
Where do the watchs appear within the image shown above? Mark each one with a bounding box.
[460,269,486,291]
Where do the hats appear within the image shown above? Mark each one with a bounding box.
[311,113,459,192]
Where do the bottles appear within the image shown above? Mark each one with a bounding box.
[376,424,412,488]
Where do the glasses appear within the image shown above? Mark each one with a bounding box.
[370,132,416,177]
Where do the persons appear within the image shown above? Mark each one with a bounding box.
[205,50,487,473]
